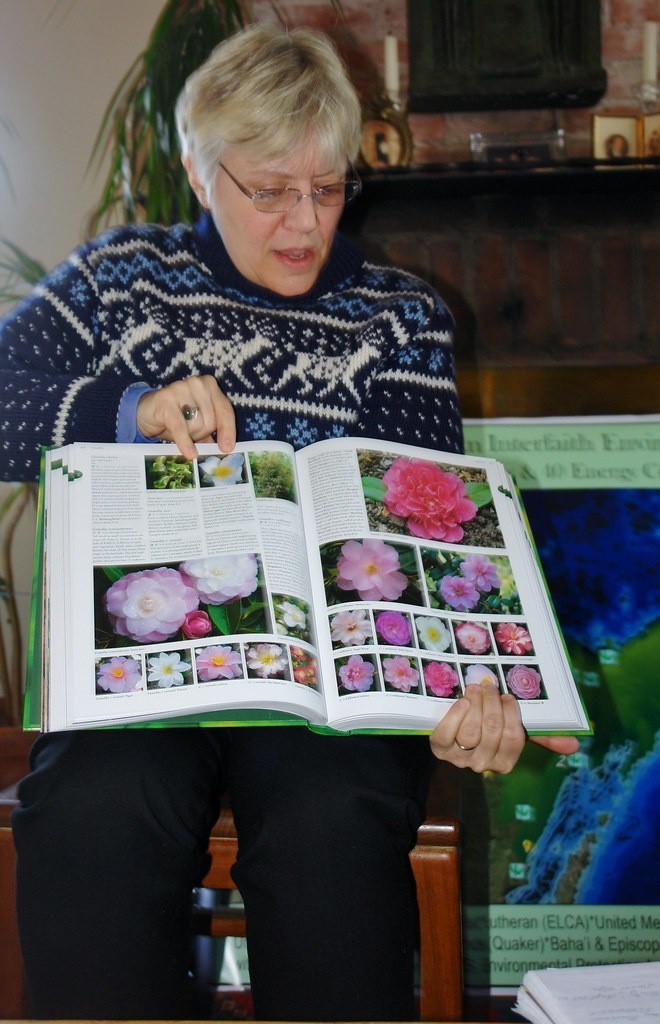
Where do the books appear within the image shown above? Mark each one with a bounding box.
[22,436,595,735]
[513,961,660,1024]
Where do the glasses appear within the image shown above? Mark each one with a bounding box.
[217,157,361,213]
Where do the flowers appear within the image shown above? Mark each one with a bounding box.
[95,452,544,700]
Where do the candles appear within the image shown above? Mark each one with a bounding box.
[642,21,658,85]
[381,32,402,92]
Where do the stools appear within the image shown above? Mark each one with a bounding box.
[0,717,459,1020]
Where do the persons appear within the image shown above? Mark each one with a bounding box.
[0,23,580,1022]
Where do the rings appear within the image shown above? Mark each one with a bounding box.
[181,405,199,420]
[455,738,476,750]
